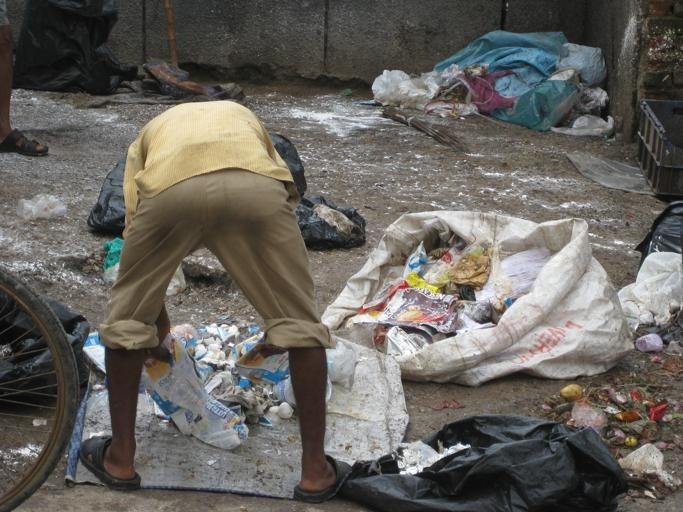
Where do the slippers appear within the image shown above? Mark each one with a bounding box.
[78,436,141,490]
[0,128,48,156]
[294,455,353,503]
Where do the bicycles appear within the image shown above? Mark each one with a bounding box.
[0,262,81,511]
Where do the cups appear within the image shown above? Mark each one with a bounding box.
[635,333,664,354]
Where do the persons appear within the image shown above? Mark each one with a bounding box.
[74,99,358,503]
[0,0,51,158]
